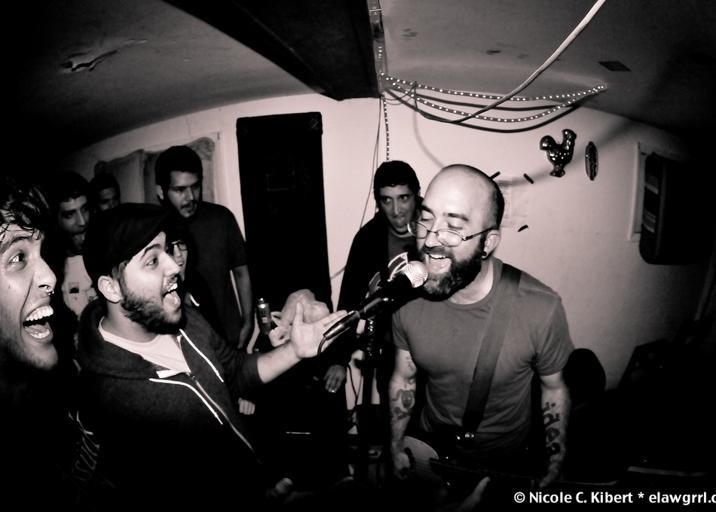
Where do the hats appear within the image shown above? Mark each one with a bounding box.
[82,201,170,277]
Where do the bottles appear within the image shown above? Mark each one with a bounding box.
[255,296,272,336]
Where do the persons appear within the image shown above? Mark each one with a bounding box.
[324,161,429,461]
[44,170,92,254]
[90,175,120,210]
[388,164,575,509]
[154,146,253,355]
[0,179,116,512]
[78,204,355,512]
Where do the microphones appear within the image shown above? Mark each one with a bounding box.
[322,261,428,338]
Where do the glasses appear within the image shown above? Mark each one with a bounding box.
[406,220,492,247]
[167,238,189,255]
